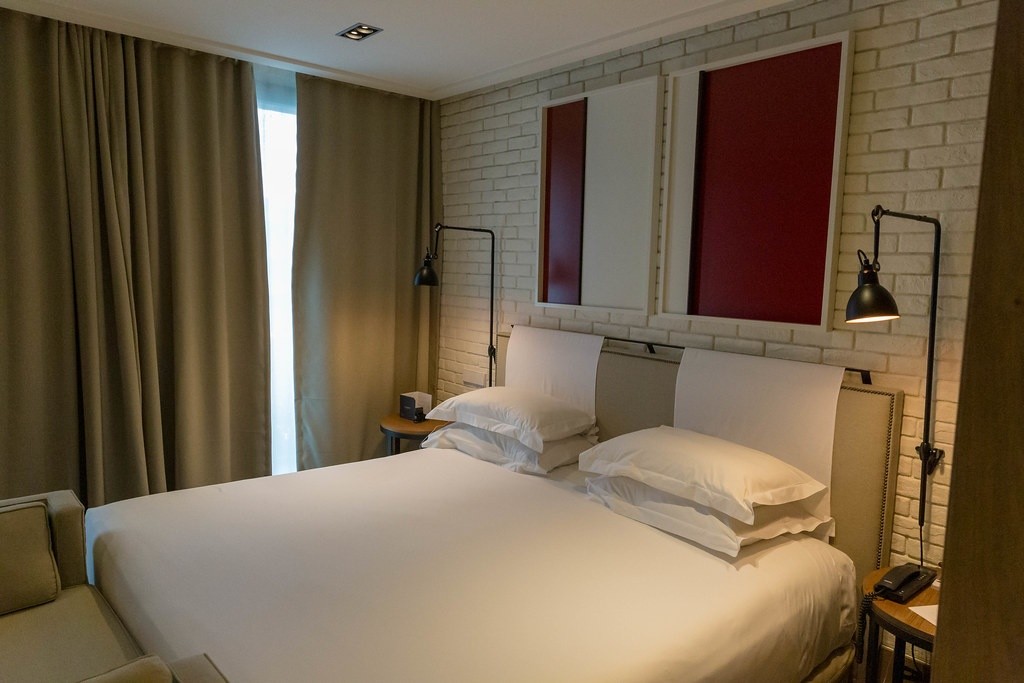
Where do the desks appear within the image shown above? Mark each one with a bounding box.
[380,411,452,455]
[856,565,938,683]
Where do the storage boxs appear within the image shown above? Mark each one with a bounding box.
[400,392,433,421]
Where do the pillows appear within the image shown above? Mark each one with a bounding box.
[586,472,818,556]
[426,383,598,451]
[0,502,62,615]
[420,419,595,474]
[577,421,826,525]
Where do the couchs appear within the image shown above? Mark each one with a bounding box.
[0,490,173,683]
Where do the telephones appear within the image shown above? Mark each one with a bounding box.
[872,562,938,604]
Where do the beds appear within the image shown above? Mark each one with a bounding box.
[86,331,898,683]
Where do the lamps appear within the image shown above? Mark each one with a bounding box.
[414,223,495,385]
[843,203,944,528]
